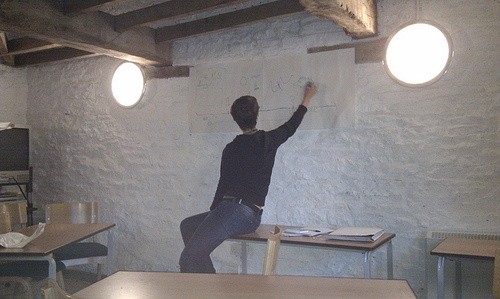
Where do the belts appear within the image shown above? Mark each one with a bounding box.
[223,197,262,215]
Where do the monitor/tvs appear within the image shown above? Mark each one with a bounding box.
[0,123,34,182]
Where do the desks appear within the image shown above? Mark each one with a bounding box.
[226,223,395,280]
[429,237,500,299]
[0,219,116,285]
[69,270,419,298]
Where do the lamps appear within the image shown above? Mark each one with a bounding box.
[382,18,454,87]
[109,60,147,110]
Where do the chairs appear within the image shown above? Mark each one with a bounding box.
[0,200,108,299]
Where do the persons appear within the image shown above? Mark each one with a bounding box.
[179,81,317,273]
